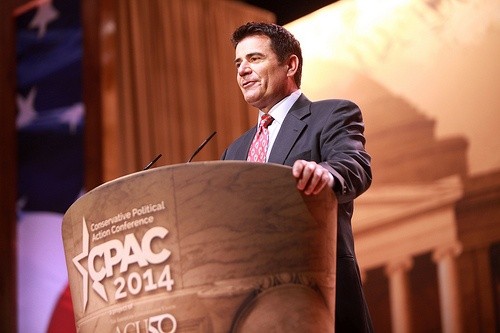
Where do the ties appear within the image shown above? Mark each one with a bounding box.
[246,114,275,163]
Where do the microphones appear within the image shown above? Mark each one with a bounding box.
[142,154,162,170]
[187,130,217,163]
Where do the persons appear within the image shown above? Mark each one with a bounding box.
[216,20,375,332]
